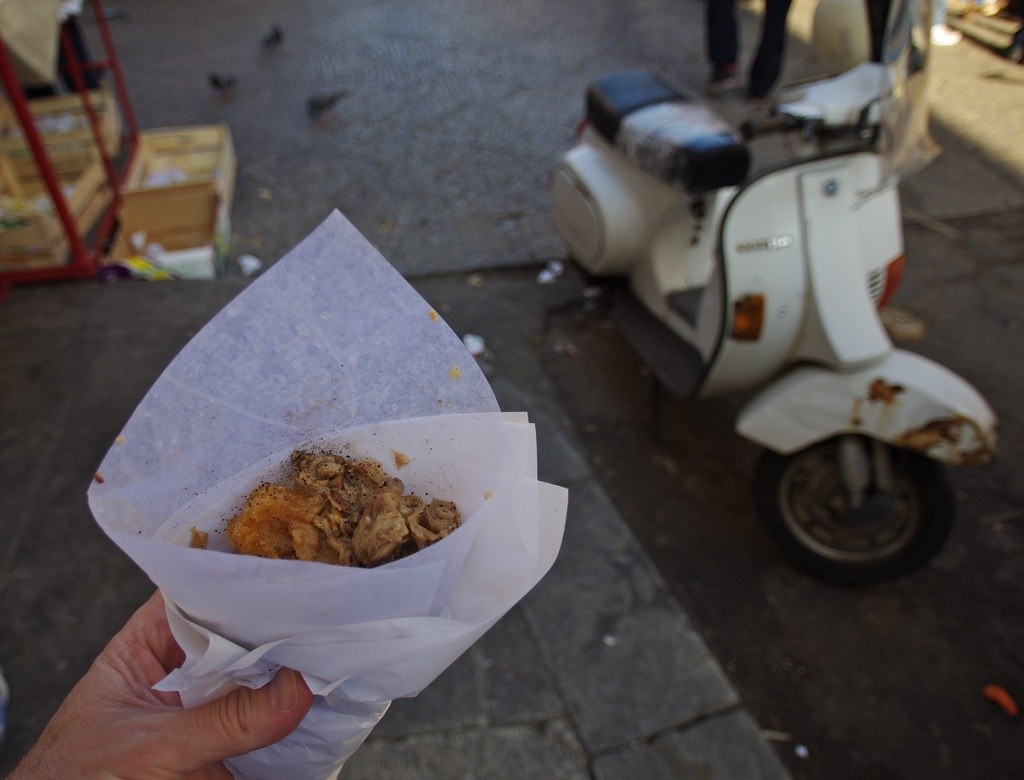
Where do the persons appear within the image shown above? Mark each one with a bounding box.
[0,584,314,780]
[701,0,794,115]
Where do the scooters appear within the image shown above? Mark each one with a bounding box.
[555,21,996,578]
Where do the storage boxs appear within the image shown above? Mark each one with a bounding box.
[1,97,234,268]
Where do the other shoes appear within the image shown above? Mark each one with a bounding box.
[705,62,736,93]
[982,0,1010,17]
[1004,41,1024,62]
[930,23,962,46]
